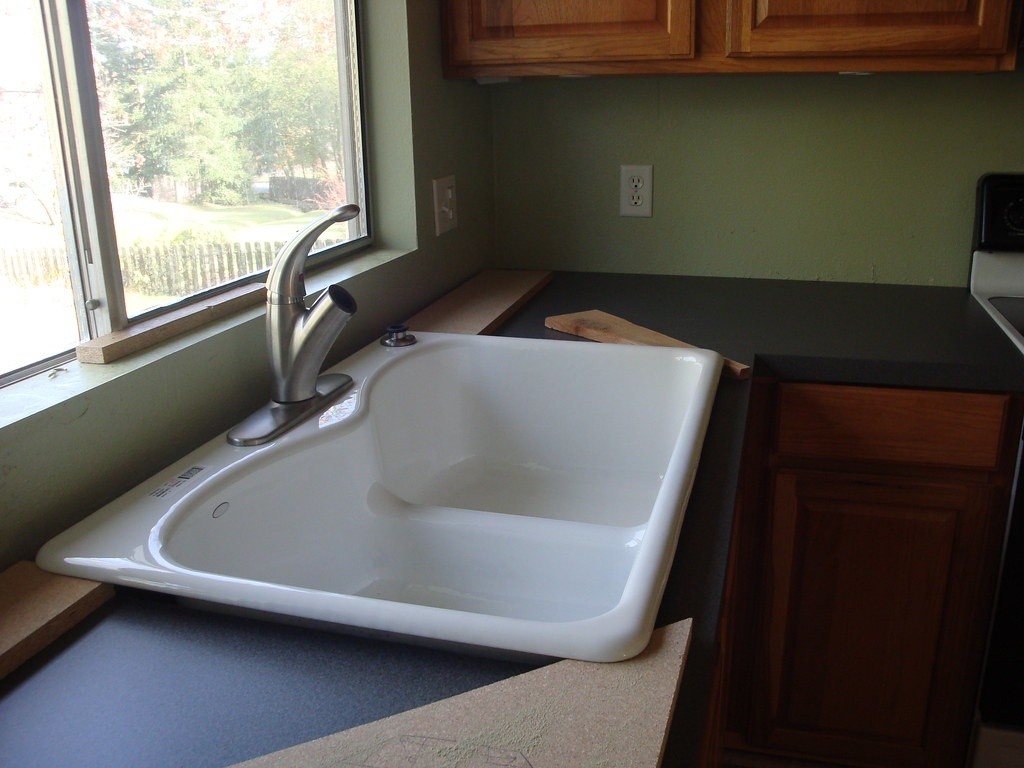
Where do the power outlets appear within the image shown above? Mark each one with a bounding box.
[619,163,654,217]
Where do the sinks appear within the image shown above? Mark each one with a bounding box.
[149,328,724,663]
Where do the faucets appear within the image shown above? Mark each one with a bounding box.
[225,204,361,448]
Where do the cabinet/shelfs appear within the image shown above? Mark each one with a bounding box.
[725,385,1024,768]
[440,1,1018,80]
